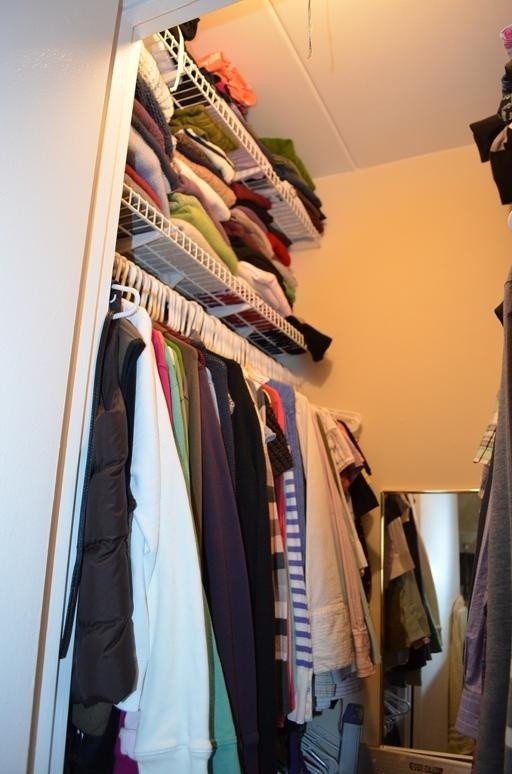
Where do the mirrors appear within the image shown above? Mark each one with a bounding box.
[369,488,483,764]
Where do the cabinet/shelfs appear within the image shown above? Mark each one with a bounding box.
[112,26,322,391]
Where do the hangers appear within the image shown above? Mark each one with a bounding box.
[113,254,304,392]
